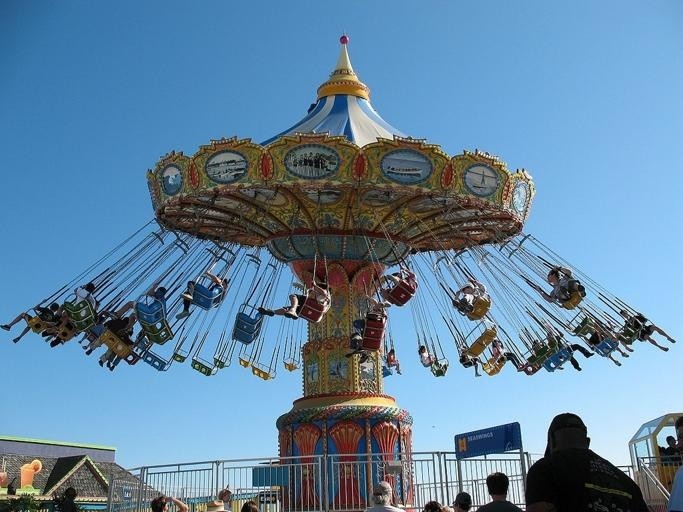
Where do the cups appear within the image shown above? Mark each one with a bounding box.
[19,464,34,488]
[0,462,7,488]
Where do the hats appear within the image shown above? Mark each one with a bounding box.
[543,411,590,458]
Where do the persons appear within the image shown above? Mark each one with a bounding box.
[345,267,447,376]
[0,270,231,372]
[61,487,78,512]
[525,413,648,511]
[150,489,260,512]
[257,275,332,323]
[452,264,676,378]
[7,477,19,496]
[657,416,683,512]
[360,472,524,512]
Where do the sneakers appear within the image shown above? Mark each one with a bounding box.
[82,345,115,372]
[284,311,299,321]
[176,292,193,320]
[0,324,20,344]
[257,307,276,317]
[577,352,594,371]
[559,285,587,299]
[451,299,473,314]
[614,337,676,366]
